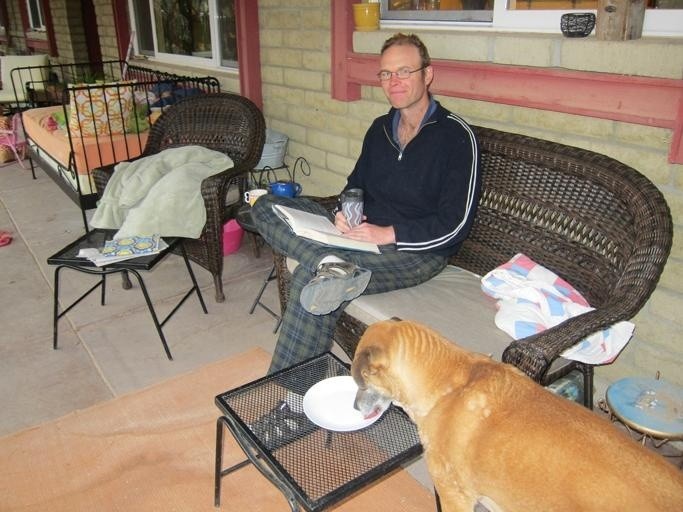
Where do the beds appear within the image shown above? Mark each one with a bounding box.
[9,59,225,239]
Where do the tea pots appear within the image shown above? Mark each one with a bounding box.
[267,179,302,198]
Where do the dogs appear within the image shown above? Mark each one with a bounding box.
[351,316,683,512]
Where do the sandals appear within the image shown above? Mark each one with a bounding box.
[245,401,312,447]
[301,263,371,315]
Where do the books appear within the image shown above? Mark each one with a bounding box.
[87,234,168,269]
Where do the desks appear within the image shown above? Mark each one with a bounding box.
[42,227,216,361]
[595,375,683,458]
[229,198,314,333]
[209,353,433,512]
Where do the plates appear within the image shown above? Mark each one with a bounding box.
[301,374,387,434]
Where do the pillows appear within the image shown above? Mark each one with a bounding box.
[65,79,135,141]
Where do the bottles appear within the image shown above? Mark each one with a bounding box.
[342,187,365,231]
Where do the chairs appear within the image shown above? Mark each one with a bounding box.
[0,112,39,171]
[90,89,264,300]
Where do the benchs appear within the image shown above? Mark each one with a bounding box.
[242,121,676,408]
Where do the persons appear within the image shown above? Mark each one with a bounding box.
[241,34,482,454]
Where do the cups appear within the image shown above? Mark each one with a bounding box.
[244,188,266,207]
[352,3,380,32]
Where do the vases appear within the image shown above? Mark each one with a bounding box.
[560,12,596,38]
[350,1,380,33]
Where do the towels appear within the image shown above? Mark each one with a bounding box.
[483,254,634,366]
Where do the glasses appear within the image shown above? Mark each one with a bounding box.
[378,68,427,80]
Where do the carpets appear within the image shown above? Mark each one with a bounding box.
[85,145,237,241]
[0,343,441,512]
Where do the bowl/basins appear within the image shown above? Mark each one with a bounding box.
[252,128,289,170]
